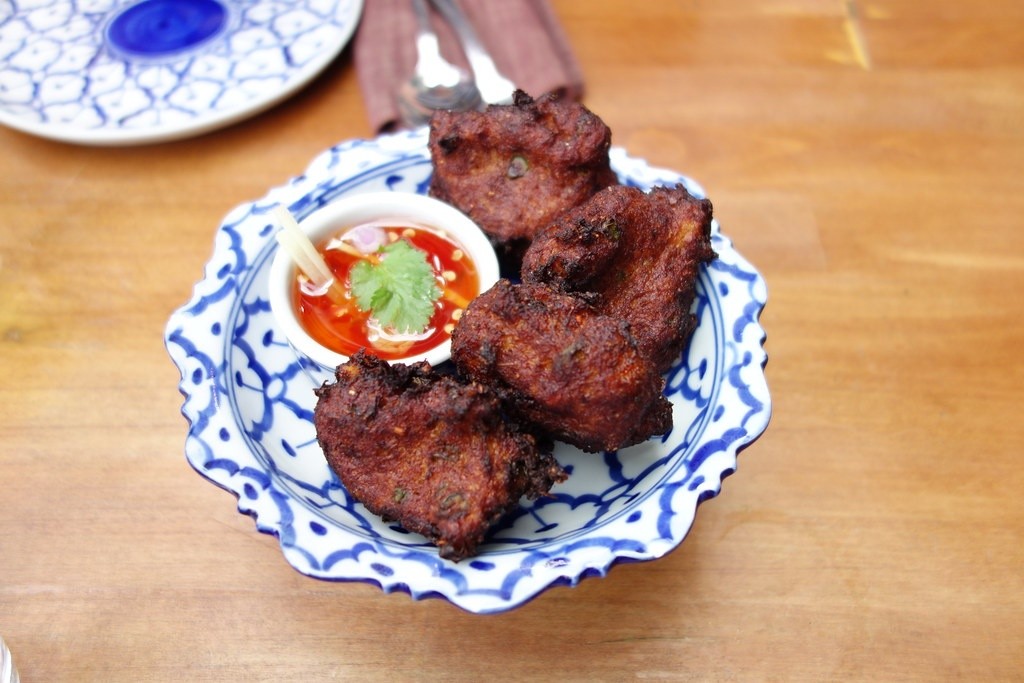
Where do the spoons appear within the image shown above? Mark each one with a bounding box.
[411,0,479,113]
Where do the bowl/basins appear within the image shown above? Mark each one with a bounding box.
[267,191,503,377]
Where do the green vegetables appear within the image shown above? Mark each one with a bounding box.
[346,237,441,335]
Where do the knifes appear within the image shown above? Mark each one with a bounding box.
[431,0,516,107]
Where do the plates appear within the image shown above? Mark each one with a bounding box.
[0,0,364,148]
[162,124,772,616]
